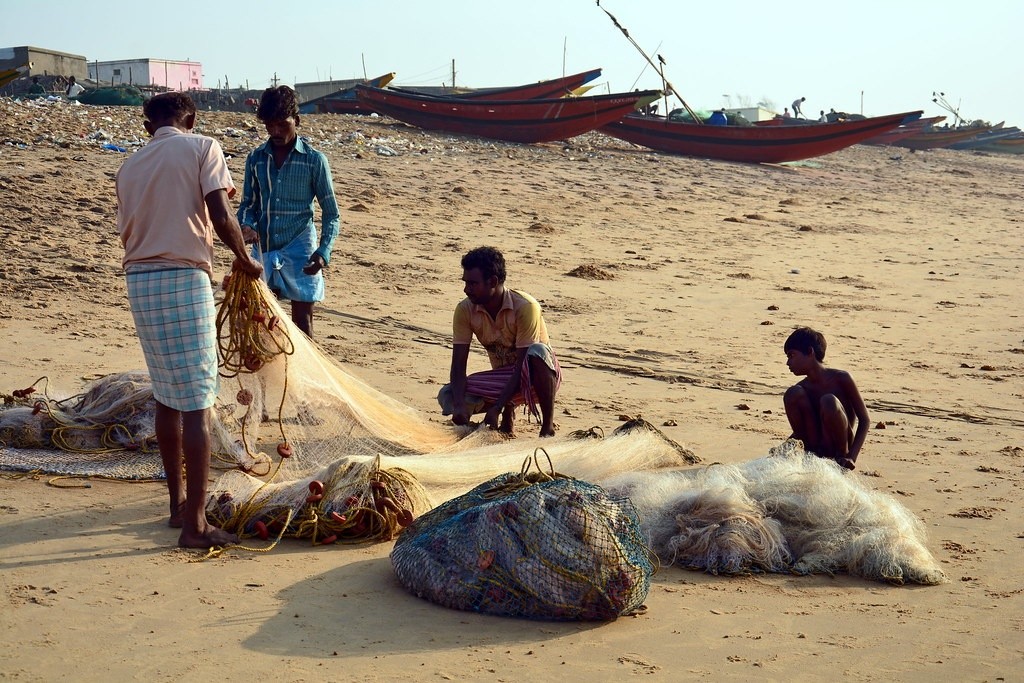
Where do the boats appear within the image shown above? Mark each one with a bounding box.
[300,73,393,113]
[318,69,1024,164]
[0,61,35,90]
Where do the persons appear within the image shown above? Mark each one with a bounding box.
[437,245,563,437]
[792,97,806,118]
[783,108,790,118]
[236,85,338,424]
[737,111,744,118]
[943,123,949,128]
[950,124,955,128]
[819,111,826,122]
[116,92,264,548]
[66,76,85,96]
[769,327,871,470]
[29,77,46,95]
[830,108,834,113]
